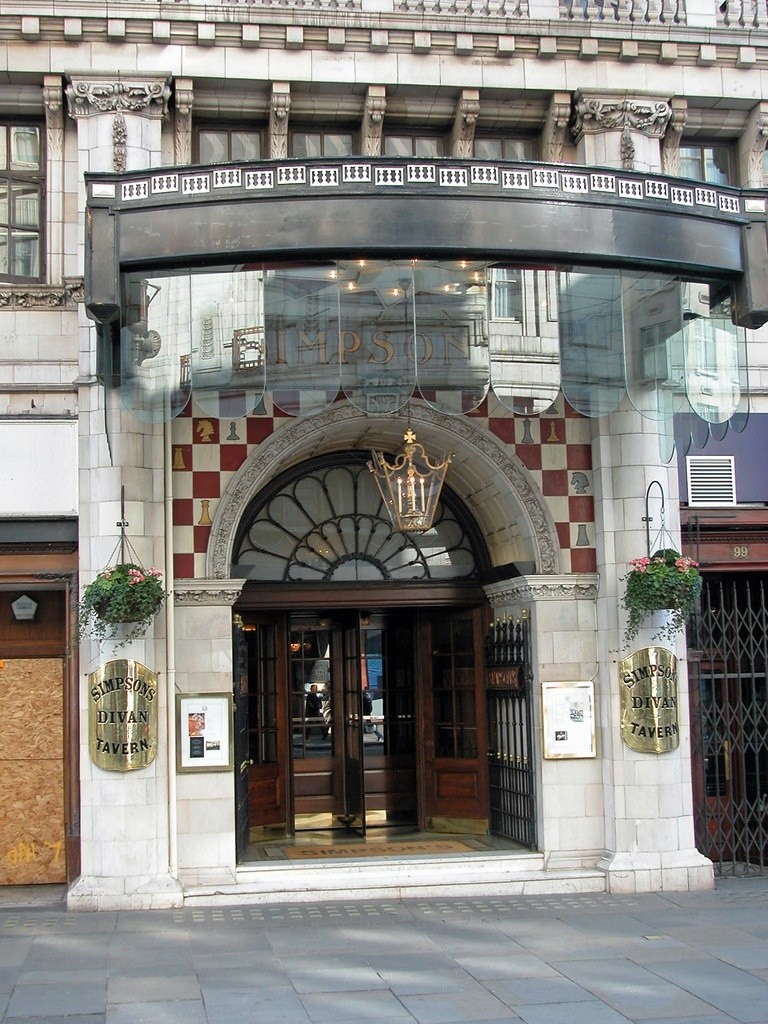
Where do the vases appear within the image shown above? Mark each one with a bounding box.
[646,596,684,610]
[93,601,155,623]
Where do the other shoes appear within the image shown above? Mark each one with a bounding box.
[377,735,383,742]
[322,733,329,740]
[305,736,309,740]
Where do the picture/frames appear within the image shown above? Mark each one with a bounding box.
[175,692,234,772]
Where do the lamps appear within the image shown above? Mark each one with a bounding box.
[366,278,453,535]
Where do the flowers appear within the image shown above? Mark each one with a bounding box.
[65,562,171,676]
[606,546,702,664]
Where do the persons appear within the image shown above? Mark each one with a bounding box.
[305,685,329,740]
[363,688,382,742]
